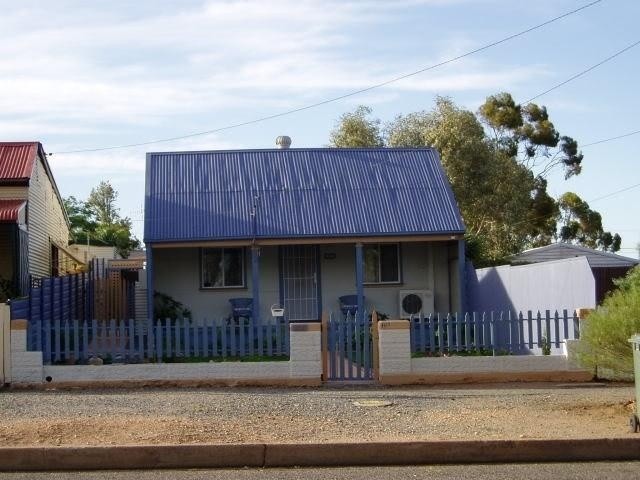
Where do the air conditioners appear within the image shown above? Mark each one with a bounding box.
[399,290,434,320]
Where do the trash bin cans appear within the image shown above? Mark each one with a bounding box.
[628,336,640,431]
